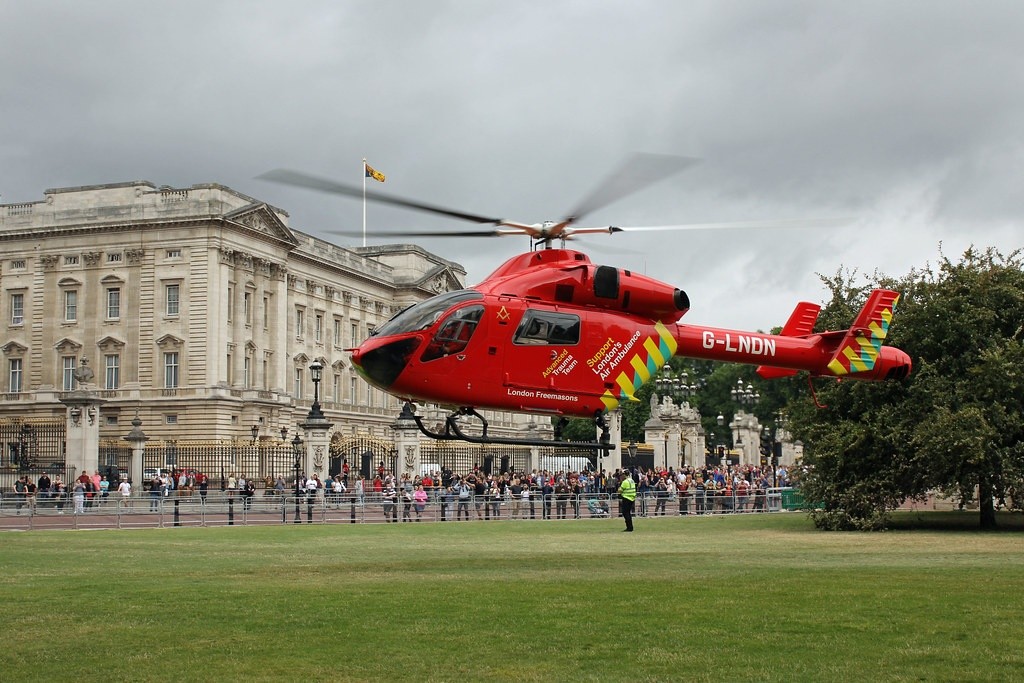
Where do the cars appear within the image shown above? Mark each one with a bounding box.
[119,466,209,488]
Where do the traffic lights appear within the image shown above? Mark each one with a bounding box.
[719,447,724,458]
[759,434,770,456]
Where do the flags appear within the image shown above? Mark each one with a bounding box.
[365,164,385,181]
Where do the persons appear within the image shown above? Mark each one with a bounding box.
[430,311,469,356]
[14,459,811,523]
[618,470,636,532]
[515,320,548,344]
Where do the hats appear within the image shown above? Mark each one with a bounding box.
[545,479,549,482]
[42,472,46,474]
[406,492,412,500]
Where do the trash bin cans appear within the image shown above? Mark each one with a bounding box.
[782,489,825,511]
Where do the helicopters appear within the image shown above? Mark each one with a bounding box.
[252,152,914,454]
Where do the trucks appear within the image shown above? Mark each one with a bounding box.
[542,457,596,473]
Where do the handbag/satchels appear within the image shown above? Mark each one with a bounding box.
[490,488,499,499]
[459,485,469,498]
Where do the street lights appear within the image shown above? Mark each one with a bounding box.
[709,432,715,466]
[717,415,724,447]
[288,433,302,524]
[655,363,790,429]
[627,439,637,480]
[307,356,326,419]
